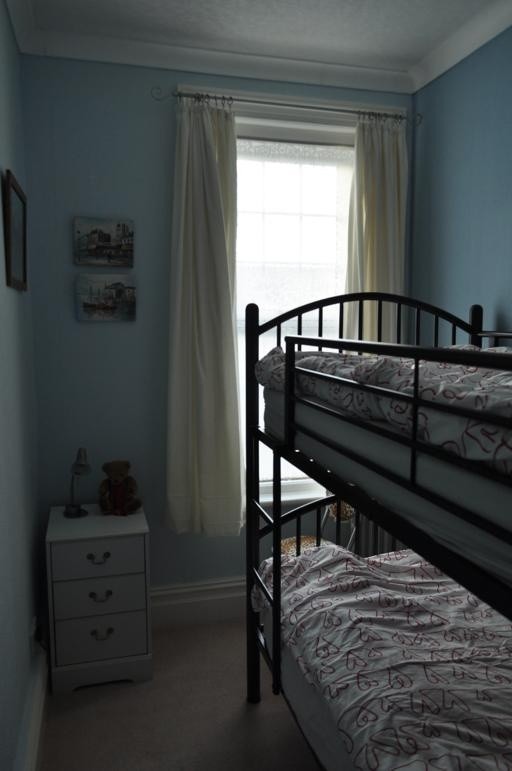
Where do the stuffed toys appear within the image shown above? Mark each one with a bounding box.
[98,460,143,516]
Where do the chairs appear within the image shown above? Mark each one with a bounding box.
[271,485,363,557]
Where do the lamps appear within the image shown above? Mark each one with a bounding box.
[63,446,92,519]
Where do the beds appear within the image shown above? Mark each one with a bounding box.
[242,293,512,771]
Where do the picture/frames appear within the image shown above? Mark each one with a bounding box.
[4,168,27,293]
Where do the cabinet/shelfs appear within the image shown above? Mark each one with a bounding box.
[45,501,152,700]
